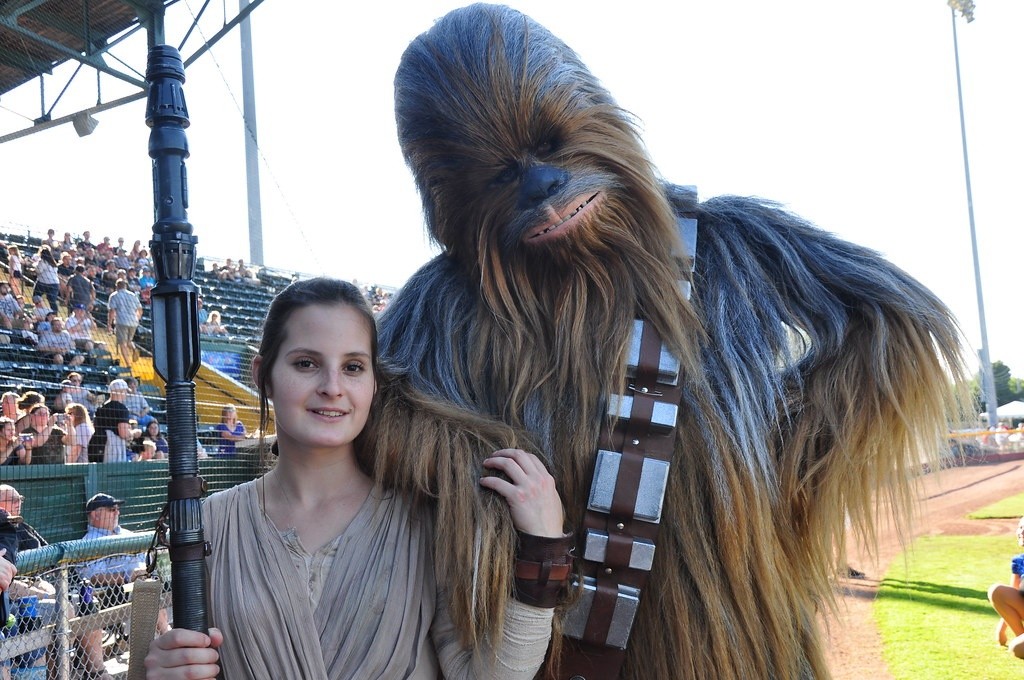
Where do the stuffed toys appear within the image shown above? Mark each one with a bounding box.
[267,2,988,680]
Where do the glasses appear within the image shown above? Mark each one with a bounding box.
[0,496,25,504]
[32,412,47,416]
[68,412,78,416]
[71,379,81,383]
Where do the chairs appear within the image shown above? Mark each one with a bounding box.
[3,232,354,609]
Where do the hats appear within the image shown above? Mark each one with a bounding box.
[75,304,86,310]
[110,379,132,393]
[86,493,125,513]
[223,403,236,412]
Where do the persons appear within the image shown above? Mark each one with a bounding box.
[143,275,572,680]
[987,516,1024,660]
[0,483,131,680]
[77,491,172,654]
[974,423,1024,448]
[0,507,20,630]
[0,229,395,467]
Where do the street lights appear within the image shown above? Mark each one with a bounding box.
[949,0,1001,431]
